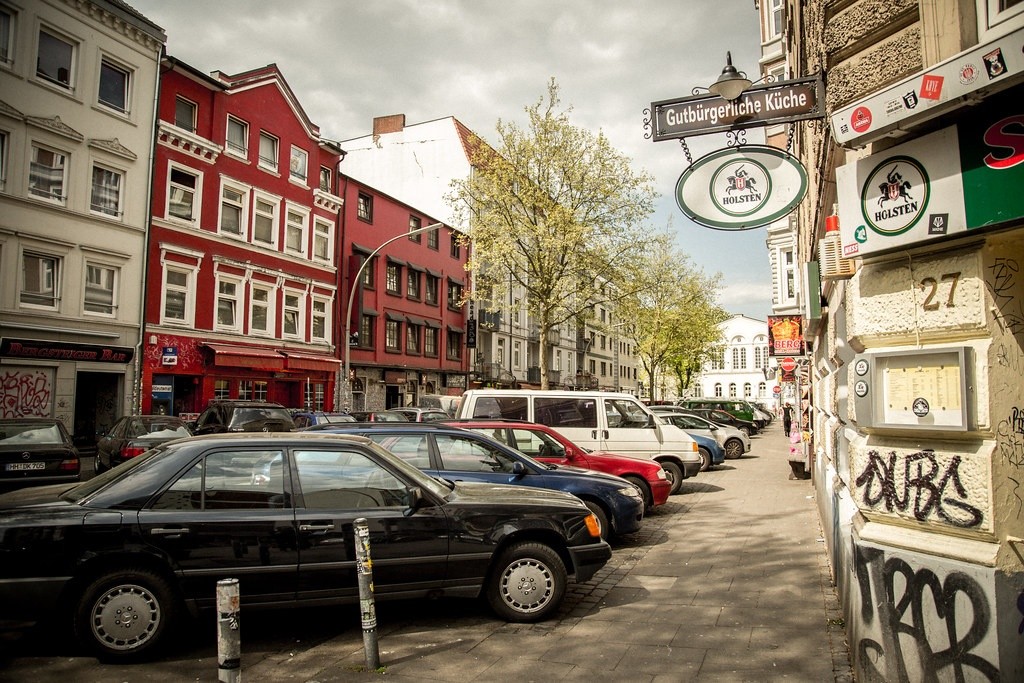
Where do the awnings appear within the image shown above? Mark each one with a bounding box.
[278,349,343,372]
[198,342,285,369]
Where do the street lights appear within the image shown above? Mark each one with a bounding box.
[343,222,445,420]
[582,322,626,390]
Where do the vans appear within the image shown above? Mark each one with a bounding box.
[449,388,704,496]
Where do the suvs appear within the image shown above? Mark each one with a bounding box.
[185,398,298,476]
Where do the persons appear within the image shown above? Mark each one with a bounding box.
[771,405,777,416]
[778,404,784,427]
[782,402,793,437]
[802,407,809,431]
[788,404,791,408]
[787,420,801,480]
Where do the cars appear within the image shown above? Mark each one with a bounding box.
[94,415,195,478]
[293,393,775,471]
[1,430,614,665]
[209,419,646,549]
[362,418,674,513]
[0,416,83,495]
